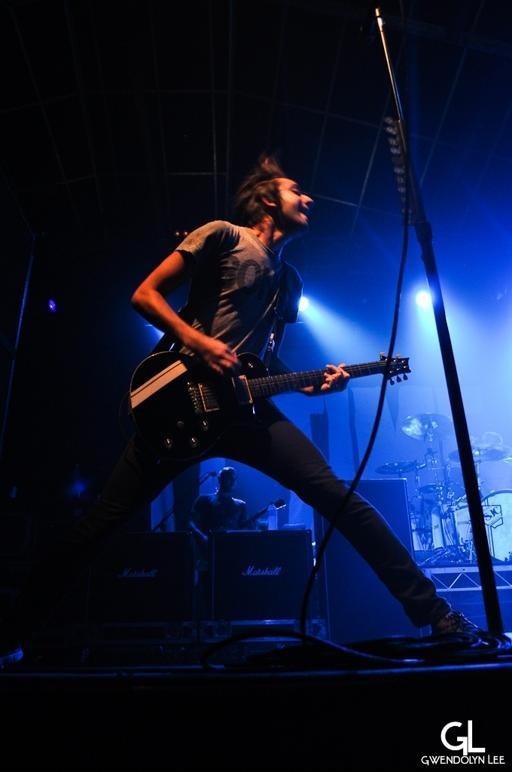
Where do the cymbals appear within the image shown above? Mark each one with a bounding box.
[448,446,510,462]
[375,459,418,474]
[401,414,454,443]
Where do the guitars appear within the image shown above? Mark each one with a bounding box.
[194,498,287,560]
[129,350,411,462]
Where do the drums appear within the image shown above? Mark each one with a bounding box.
[413,460,448,497]
[468,488,512,565]
[443,461,466,499]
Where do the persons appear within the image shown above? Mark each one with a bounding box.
[1,150,499,654]
[187,465,258,636]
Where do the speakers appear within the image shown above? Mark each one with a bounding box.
[313,477,423,643]
[98,530,194,628]
[204,528,314,631]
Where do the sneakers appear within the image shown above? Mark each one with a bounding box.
[431,608,483,637]
